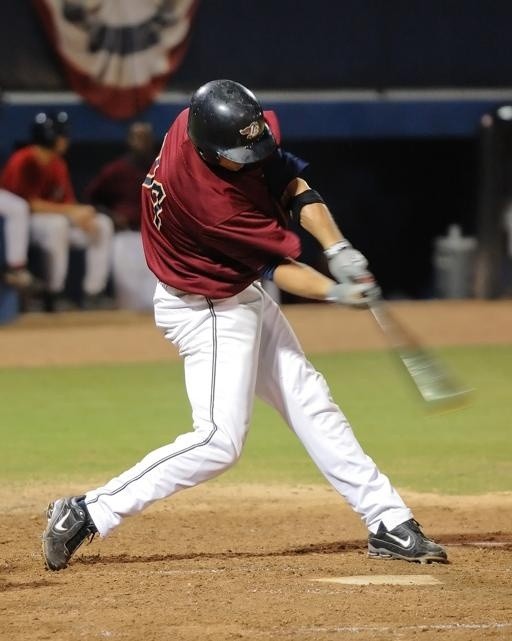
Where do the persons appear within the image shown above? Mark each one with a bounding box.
[42,79,448,571]
[0,120,159,310]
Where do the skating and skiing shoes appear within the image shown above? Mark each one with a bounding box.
[2,266,117,311]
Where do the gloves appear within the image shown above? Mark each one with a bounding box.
[323,240,382,309]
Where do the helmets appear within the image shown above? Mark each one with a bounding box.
[188,79,277,162]
[33,111,72,149]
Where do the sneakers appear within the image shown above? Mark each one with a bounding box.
[43,495,96,571]
[369,518,448,563]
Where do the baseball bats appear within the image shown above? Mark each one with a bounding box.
[362,291,477,413]
